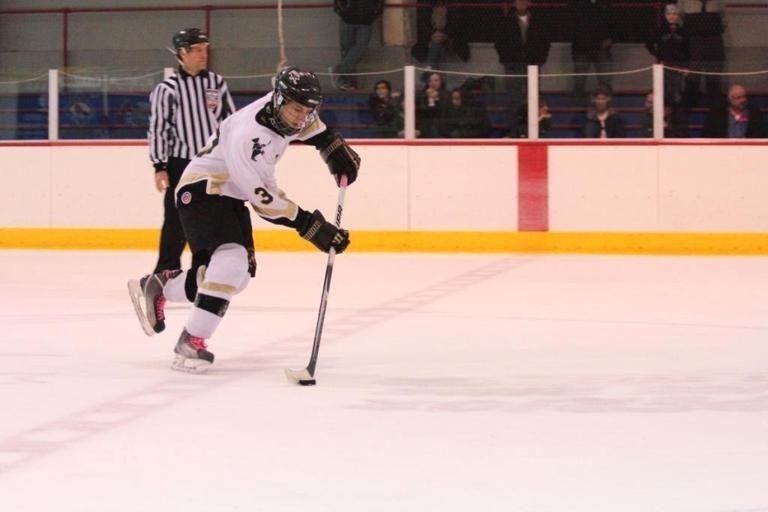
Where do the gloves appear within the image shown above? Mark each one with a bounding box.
[319,133,361,187]
[299,210,350,254]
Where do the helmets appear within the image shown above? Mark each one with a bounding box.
[174,27,210,53]
[275,67,321,135]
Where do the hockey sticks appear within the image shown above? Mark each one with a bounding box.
[283,172,348,380]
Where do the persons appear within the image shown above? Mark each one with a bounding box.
[413,0,727,97]
[147,28,238,276]
[127,66,361,374]
[330,0,382,92]
[507,96,552,139]
[371,71,477,138]
[583,86,625,138]
[703,83,767,138]
[639,92,689,138]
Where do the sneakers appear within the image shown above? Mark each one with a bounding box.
[140,273,168,333]
[174,330,213,362]
